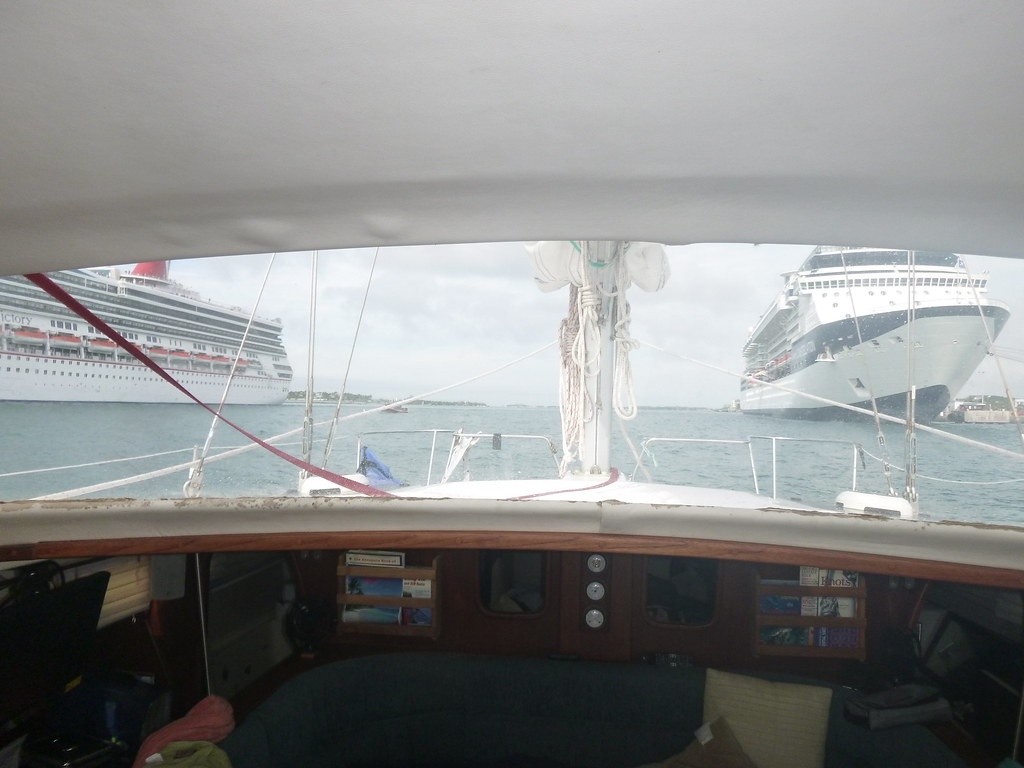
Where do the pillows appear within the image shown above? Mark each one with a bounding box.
[636,668,833,768]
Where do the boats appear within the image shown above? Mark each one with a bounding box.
[10,324,248,371]
[947,403,986,423]
[381,398,408,413]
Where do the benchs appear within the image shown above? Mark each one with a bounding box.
[216,647,974,768]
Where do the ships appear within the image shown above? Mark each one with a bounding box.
[0,259,293,406]
[739,247,1011,425]
